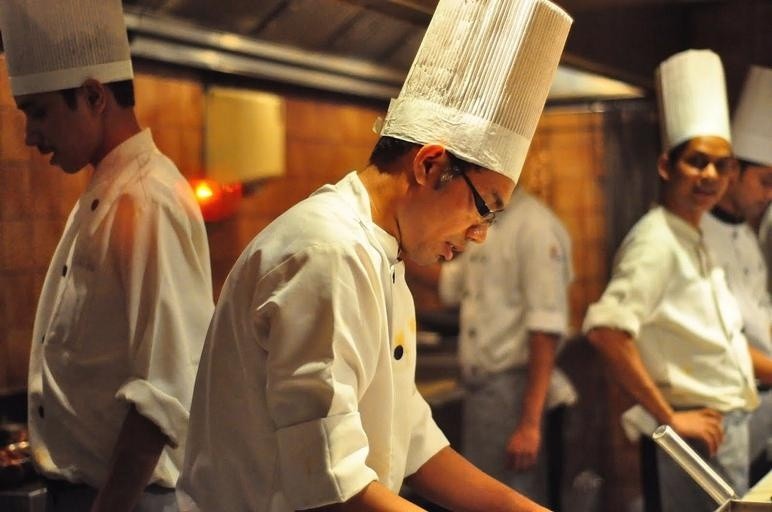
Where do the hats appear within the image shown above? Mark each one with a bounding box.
[379,0,573,185]
[731,64,772,168]
[654,47,732,151]
[1,0,134,97]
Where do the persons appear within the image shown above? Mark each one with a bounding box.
[173,1,576,511]
[0,0,218,510]
[697,62,772,487]
[437,185,577,512]
[578,47,761,511]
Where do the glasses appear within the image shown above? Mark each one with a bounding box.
[448,152,494,223]
